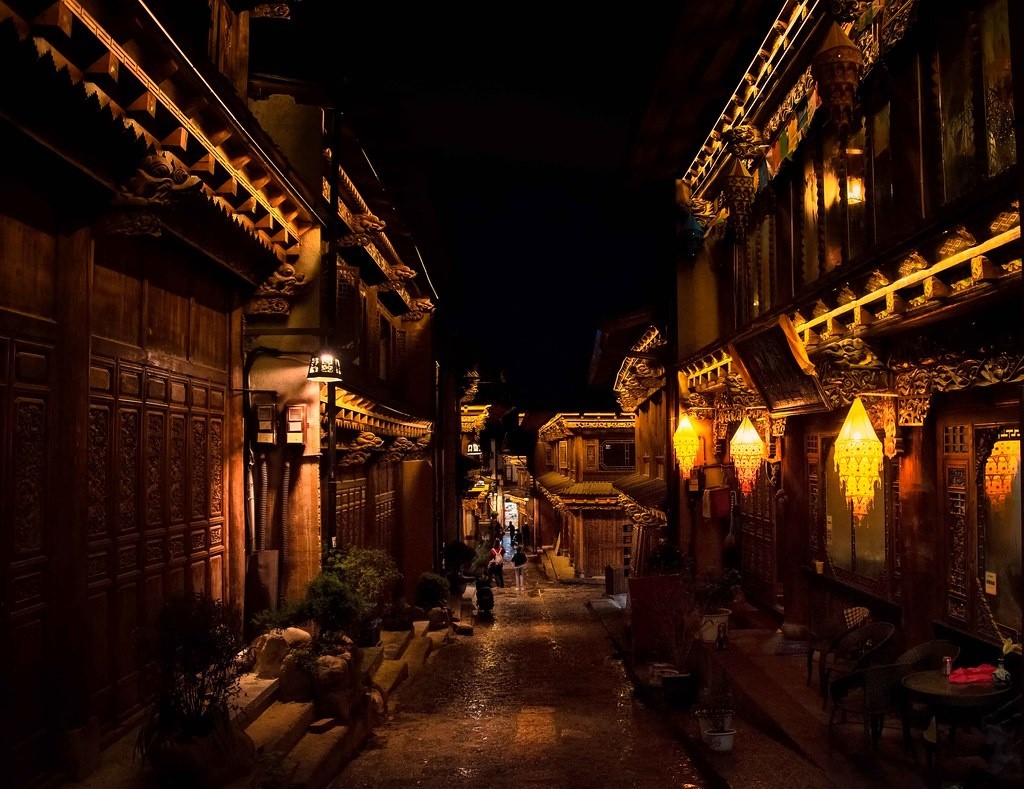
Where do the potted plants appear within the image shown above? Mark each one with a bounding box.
[247,573,355,662]
[135,588,254,781]
[704,705,737,751]
[416,572,450,609]
[660,611,698,713]
[333,543,404,647]
[694,679,737,744]
[694,568,737,643]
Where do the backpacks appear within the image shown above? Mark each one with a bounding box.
[492,548,504,565]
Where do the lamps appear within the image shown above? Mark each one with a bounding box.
[248,328,343,382]
[672,407,714,479]
[833,393,902,528]
[729,406,768,498]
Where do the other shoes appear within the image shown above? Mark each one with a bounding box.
[515,587,519,590]
[521,587,525,590]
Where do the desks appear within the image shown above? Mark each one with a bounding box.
[900,669,1011,758]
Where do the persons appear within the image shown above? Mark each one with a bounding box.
[511,546,528,591]
[490,539,506,588]
[489,521,530,548]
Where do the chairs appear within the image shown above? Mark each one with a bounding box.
[806,606,962,760]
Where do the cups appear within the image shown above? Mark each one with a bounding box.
[816,561,824,574]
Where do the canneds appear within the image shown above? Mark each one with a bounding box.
[941,656,952,675]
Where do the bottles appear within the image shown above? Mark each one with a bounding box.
[993,658,1010,687]
